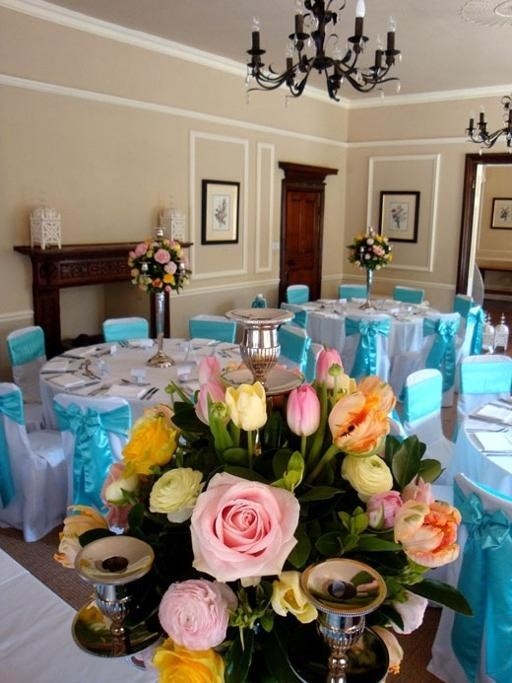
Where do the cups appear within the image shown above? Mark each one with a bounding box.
[399,301,413,319]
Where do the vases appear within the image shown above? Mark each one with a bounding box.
[359,269,377,310]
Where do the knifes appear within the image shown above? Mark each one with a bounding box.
[141,387,155,400]
[146,388,159,400]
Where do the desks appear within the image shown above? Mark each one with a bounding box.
[13,240,193,361]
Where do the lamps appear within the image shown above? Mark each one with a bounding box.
[465,92,512,156]
[247,0,402,101]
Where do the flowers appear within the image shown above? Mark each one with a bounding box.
[345,227,392,273]
[127,239,192,294]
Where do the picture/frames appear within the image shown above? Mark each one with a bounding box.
[490,198,512,229]
[202,179,240,244]
[379,191,420,242]
